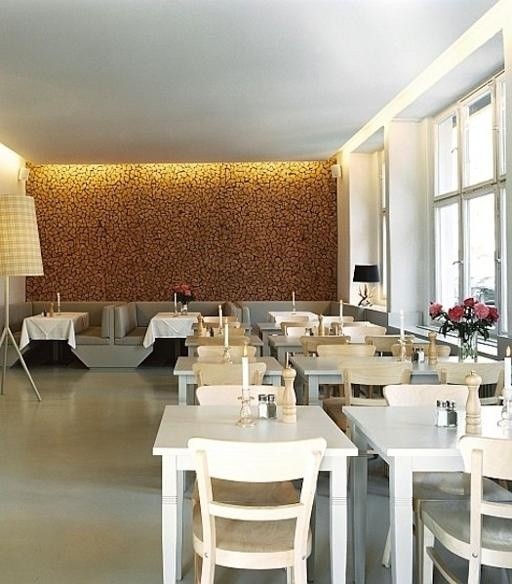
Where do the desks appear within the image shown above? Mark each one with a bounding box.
[144,311,200,366]
[21,312,89,367]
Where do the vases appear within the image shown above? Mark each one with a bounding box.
[181,301,188,315]
[458,328,478,363]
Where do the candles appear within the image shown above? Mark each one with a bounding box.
[340,300,342,322]
[174,293,177,310]
[225,318,228,348]
[504,345,511,395]
[399,309,404,340]
[292,291,295,308]
[57,293,60,310]
[219,304,222,329]
[242,344,250,398]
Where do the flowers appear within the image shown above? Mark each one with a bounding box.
[171,284,198,304]
[428,296,498,359]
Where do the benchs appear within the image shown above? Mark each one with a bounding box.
[70,302,112,368]
[0,301,32,367]
[112,302,154,368]
[32,301,114,326]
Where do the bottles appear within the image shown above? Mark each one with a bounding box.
[304,328,314,336]
[412,348,425,363]
[446,400,457,428]
[257,394,268,420]
[41,309,48,318]
[435,400,447,427]
[267,394,276,419]
[206,327,215,337]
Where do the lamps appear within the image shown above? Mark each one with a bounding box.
[353,265,380,306]
[0,194,45,402]
